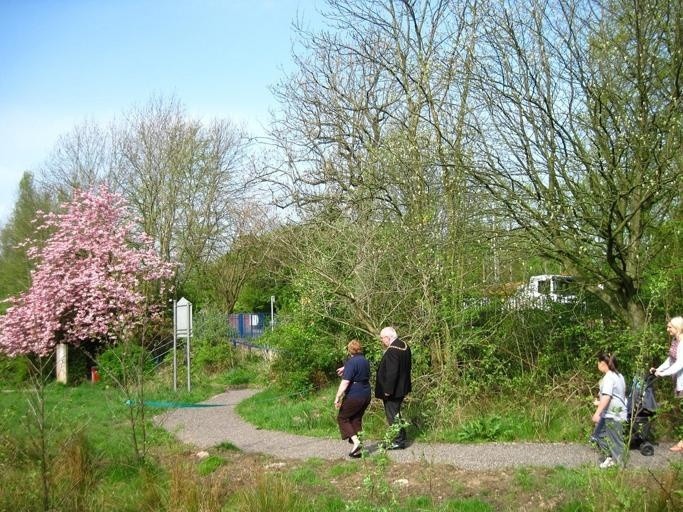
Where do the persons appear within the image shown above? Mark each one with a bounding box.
[375,327,412,450]
[334,340,371,458]
[592,353,628,469]
[650,316,683,453]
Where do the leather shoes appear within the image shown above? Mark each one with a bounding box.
[376,439,406,451]
[669,437,683,453]
[348,444,363,458]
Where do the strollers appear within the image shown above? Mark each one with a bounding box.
[589,369,664,458]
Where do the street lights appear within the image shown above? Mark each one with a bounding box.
[268,292,279,332]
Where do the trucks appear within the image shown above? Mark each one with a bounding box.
[511,271,606,306]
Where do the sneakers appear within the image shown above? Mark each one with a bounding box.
[599,456,617,468]
